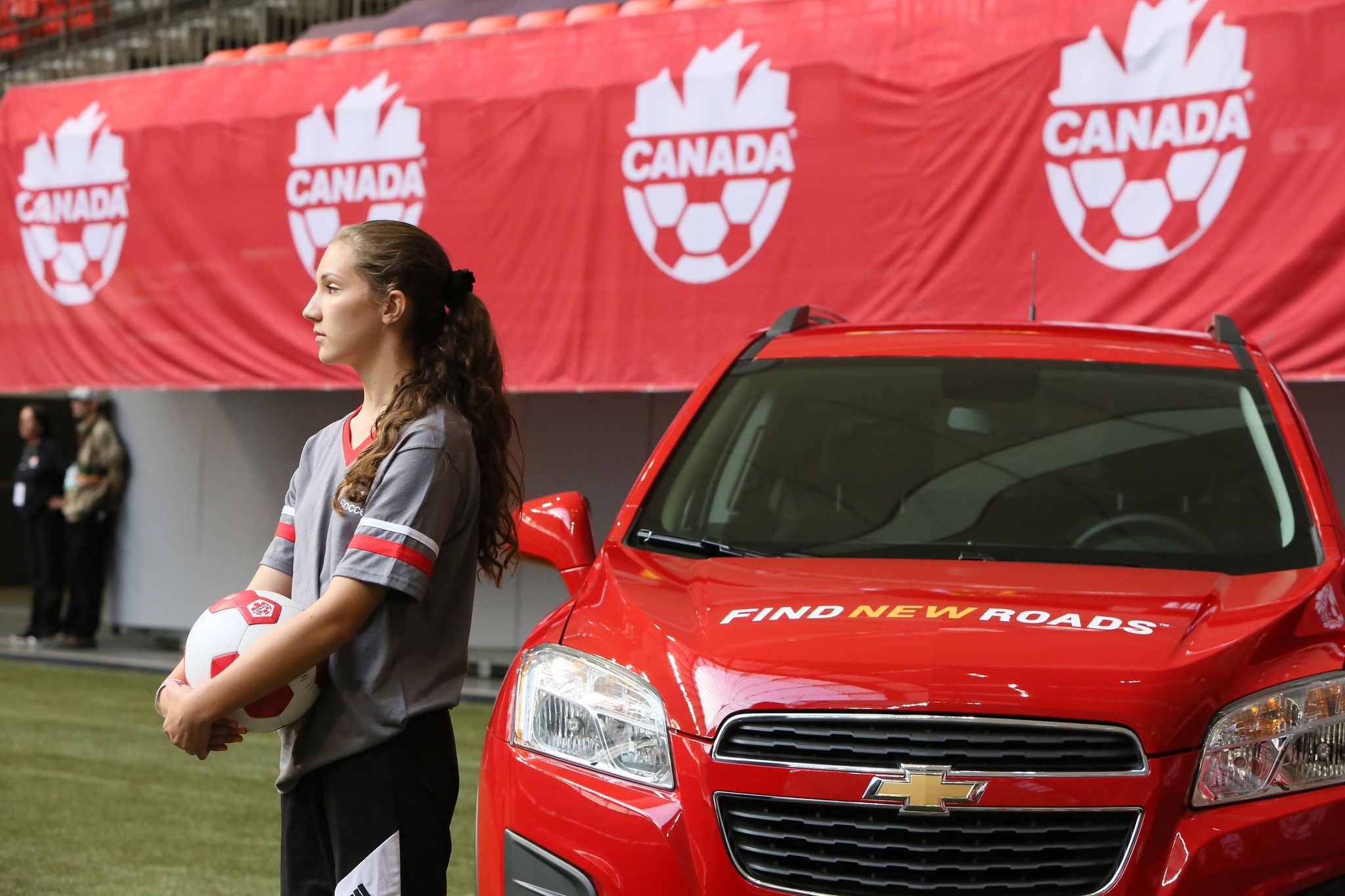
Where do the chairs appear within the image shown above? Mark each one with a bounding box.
[0,0,109,54]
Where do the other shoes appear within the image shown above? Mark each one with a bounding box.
[64,634,98,651]
[7,632,66,649]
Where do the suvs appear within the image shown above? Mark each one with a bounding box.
[473,303,1343,896]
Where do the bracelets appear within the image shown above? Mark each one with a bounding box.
[155,679,188,718]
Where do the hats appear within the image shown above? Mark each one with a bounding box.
[67,386,103,405]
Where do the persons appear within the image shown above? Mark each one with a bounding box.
[2,387,131,652]
[154,219,527,896]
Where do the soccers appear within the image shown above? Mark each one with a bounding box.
[179,587,323,734]
